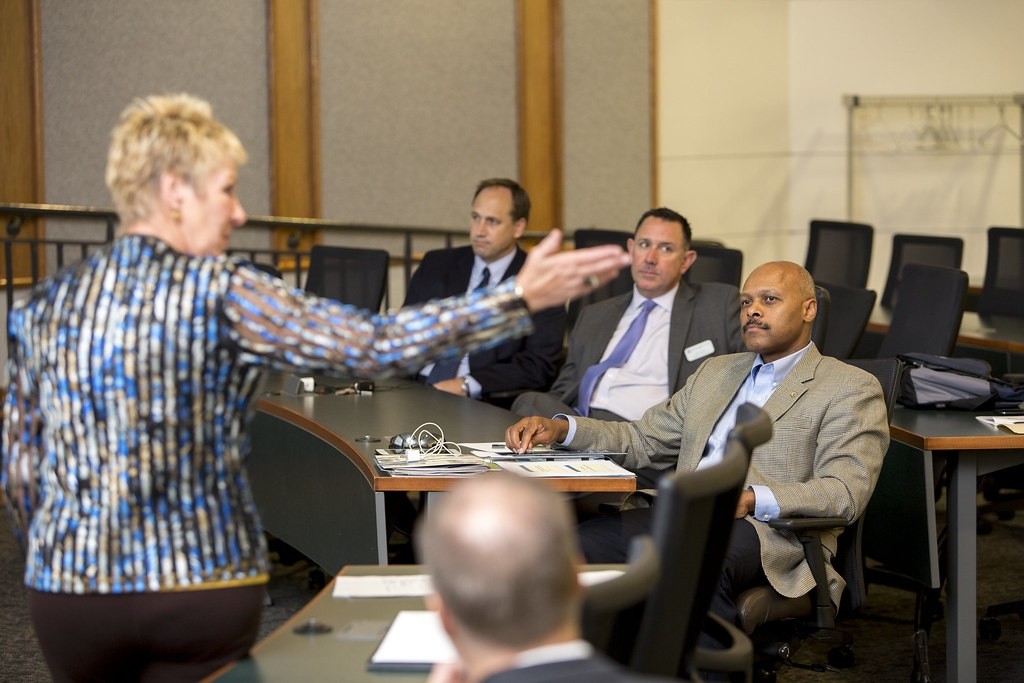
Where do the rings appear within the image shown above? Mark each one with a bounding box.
[588,274,600,288]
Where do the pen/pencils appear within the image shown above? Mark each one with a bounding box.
[491,443,552,450]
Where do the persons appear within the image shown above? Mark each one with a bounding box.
[413,468,676,683]
[0,94,632,683]
[504,261,891,622]
[397,177,568,408]
[512,207,744,491]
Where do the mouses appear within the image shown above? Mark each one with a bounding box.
[390,432,418,448]
[419,432,448,450]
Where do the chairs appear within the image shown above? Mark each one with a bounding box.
[234,220,1024,683]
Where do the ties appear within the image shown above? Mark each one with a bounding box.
[425,268,491,385]
[573,300,656,418]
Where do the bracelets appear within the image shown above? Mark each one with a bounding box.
[462,377,470,398]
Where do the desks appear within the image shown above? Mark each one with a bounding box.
[879,402,1024,683]
[196,565,427,683]
[243,373,637,578]
[865,297,1024,375]
